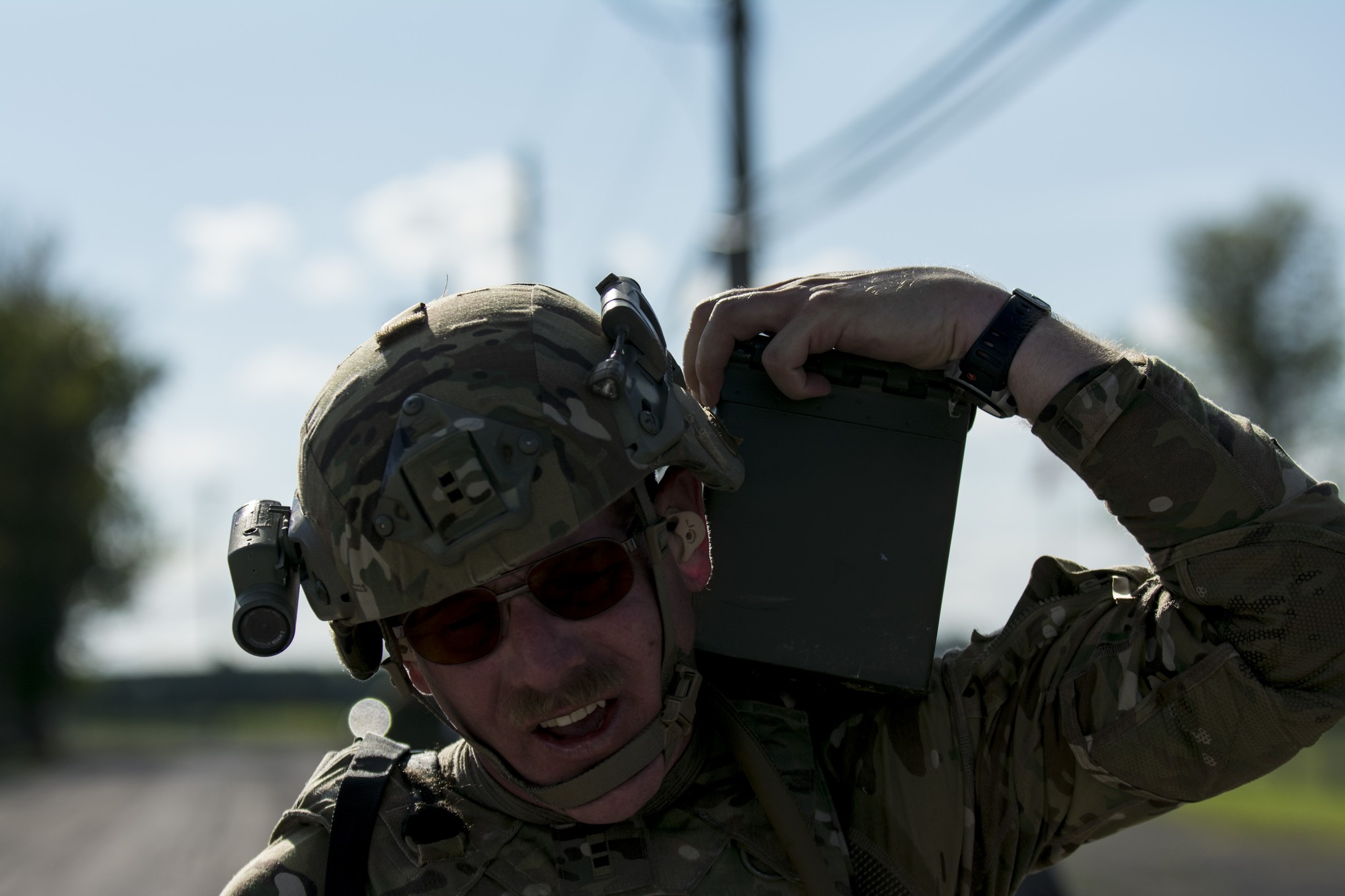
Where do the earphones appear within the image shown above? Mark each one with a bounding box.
[663,510,706,560]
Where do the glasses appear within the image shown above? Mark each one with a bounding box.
[363,516,668,664]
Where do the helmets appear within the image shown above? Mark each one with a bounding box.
[294,279,760,681]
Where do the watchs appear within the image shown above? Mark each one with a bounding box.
[937,285,1051,420]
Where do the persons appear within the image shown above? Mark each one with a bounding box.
[215,261,1344,896]
